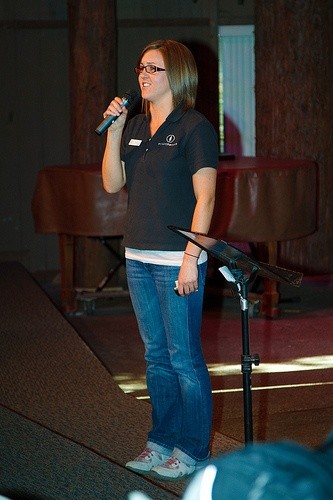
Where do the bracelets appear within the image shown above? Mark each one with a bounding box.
[184,252,199,259]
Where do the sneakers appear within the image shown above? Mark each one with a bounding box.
[150,455,210,482]
[125,448,171,475]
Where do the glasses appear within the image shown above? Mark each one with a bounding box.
[134,62,166,74]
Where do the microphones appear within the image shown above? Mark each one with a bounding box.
[94,86,140,136]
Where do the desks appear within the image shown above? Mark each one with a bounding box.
[31,159,317,317]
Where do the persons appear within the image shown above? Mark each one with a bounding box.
[183,436,333,500]
[101,39,221,484]
